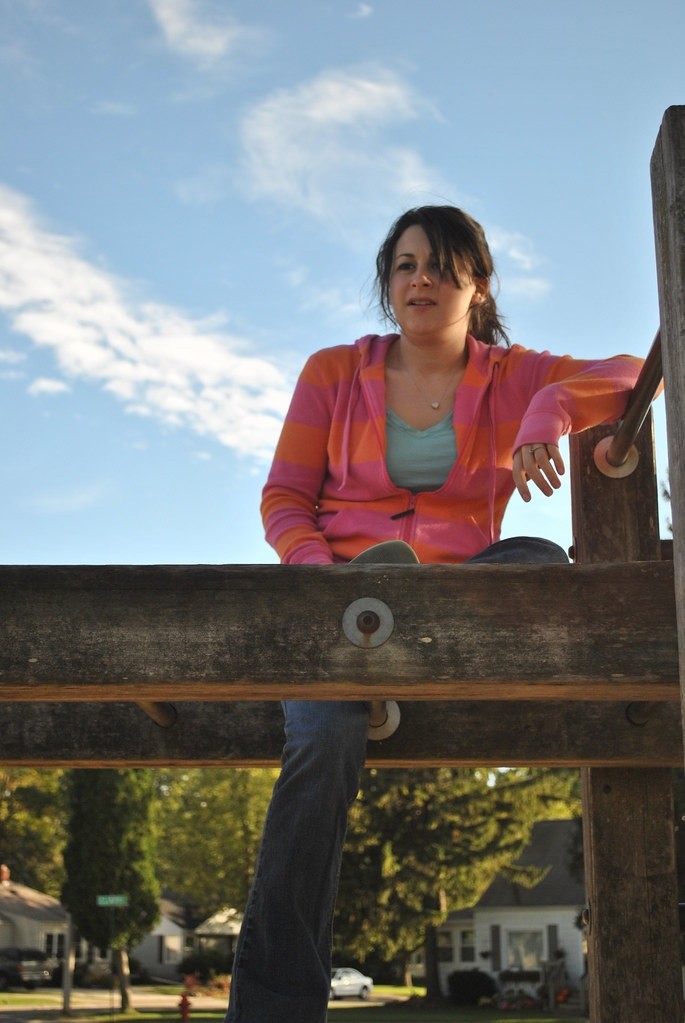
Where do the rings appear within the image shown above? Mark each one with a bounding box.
[529,446,544,453]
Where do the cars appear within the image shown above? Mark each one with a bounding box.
[0,944,54,989]
[327,966,374,1002]
[500,966,546,1008]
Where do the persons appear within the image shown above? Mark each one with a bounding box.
[227,206,665,1022]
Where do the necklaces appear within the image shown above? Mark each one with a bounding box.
[399,356,458,409]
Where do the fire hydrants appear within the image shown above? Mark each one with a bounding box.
[177,993,191,1023]
